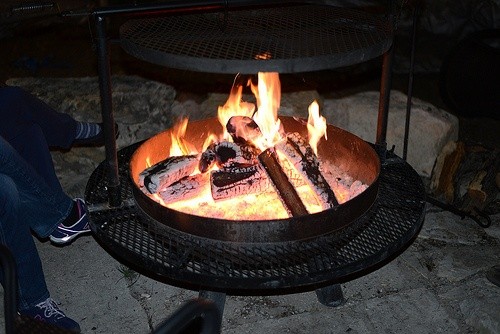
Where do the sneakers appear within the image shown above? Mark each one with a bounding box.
[18,298,81,334]
[48,197,108,245]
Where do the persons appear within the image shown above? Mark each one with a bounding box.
[0,84,121,197]
[0,136,92,334]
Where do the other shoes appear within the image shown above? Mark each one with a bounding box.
[72,120,119,146]
